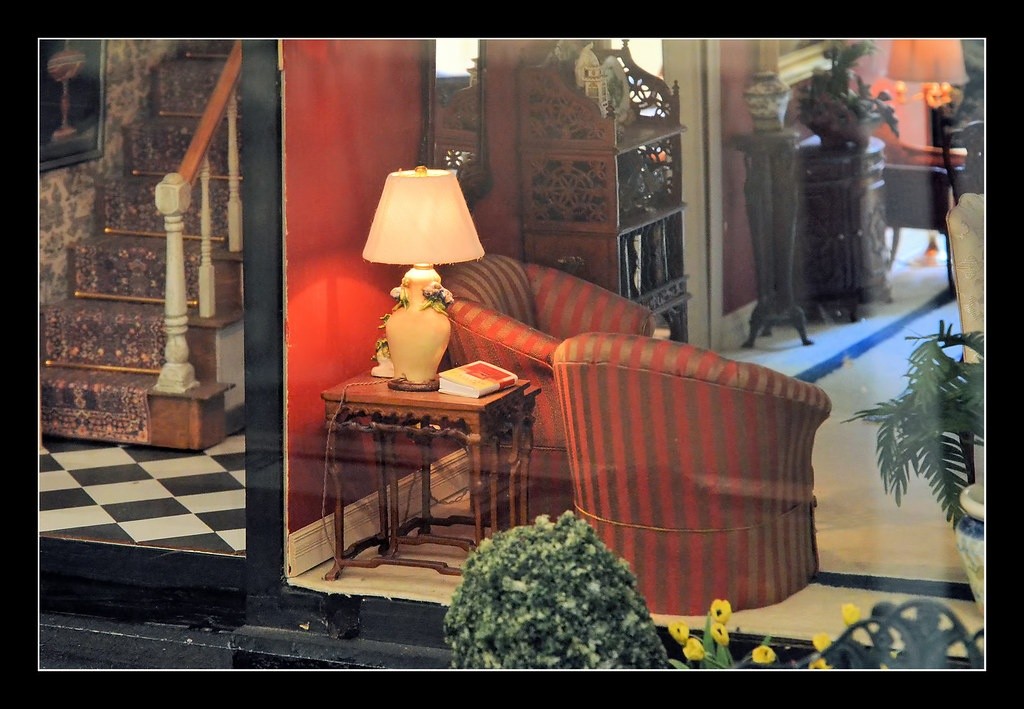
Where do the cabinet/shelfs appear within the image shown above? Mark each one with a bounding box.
[511,43,689,347]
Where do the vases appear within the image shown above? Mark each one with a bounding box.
[742,72,791,130]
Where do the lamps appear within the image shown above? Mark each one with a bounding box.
[889,38,970,154]
[361,166,487,385]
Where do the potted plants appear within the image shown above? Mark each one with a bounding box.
[853,324,985,616]
[800,42,903,156]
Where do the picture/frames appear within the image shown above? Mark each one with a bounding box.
[38,41,105,171]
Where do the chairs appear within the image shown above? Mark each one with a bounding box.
[878,163,955,291]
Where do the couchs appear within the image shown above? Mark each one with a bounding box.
[444,252,682,497]
[553,330,837,617]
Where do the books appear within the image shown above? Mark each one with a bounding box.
[438,360,519,397]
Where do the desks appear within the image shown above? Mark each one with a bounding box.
[317,366,540,579]
